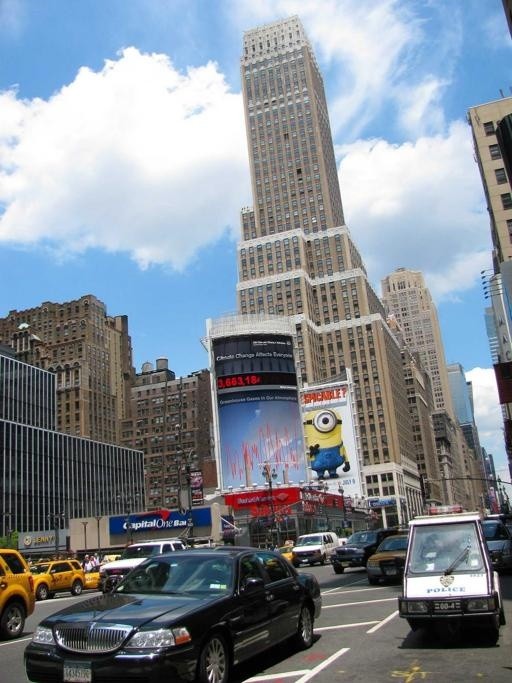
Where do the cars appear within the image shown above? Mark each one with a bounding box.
[24,546,323,683]
[0,539,185,640]
[279,505,511,634]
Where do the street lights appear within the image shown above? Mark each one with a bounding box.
[262,460,278,547]
[116,493,140,546]
[94,516,103,552]
[1,511,15,540]
[80,519,88,553]
[176,444,198,537]
[46,512,65,559]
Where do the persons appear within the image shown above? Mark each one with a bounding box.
[27,555,99,572]
